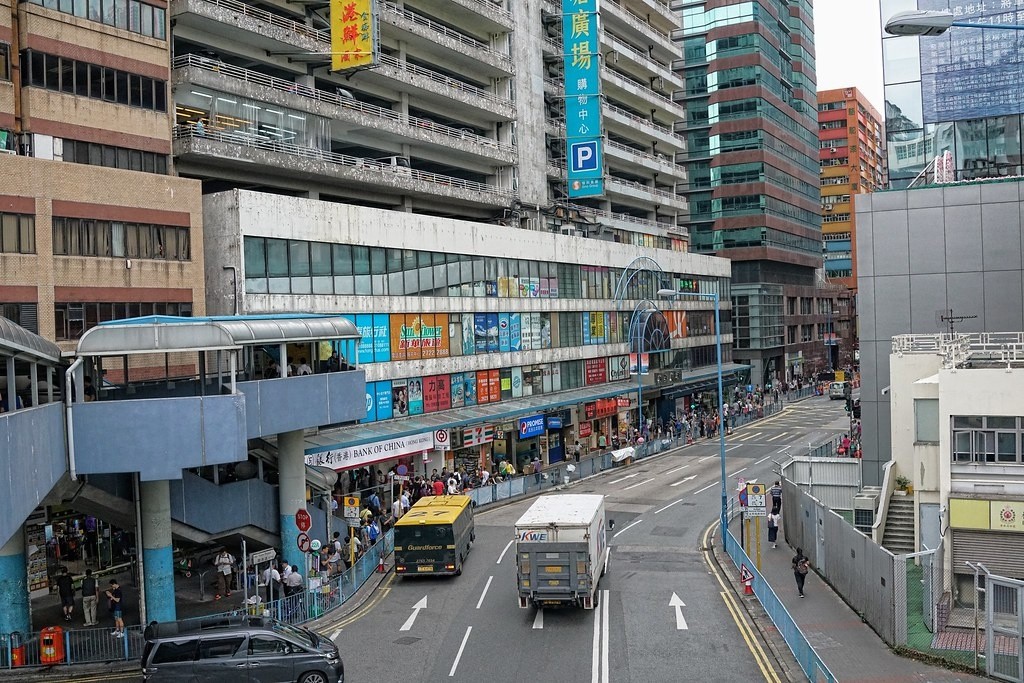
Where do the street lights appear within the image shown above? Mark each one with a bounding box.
[827,310,840,372]
[636,307,658,439]
[656,287,728,551]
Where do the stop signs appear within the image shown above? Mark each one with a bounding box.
[294,508,313,533]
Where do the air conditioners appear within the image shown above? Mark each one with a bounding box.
[822,242,826,249]
[825,204,832,210]
[560,224,575,235]
[821,205,824,208]
[820,168,824,173]
[830,148,837,153]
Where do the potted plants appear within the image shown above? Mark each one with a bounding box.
[892,475,913,496]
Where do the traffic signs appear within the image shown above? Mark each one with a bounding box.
[251,549,277,566]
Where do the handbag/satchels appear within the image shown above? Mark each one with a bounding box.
[268,578,280,592]
[767,518,774,528]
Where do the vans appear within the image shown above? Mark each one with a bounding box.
[138,614,345,683]
[828,381,852,400]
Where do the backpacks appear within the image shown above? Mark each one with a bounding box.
[341,544,350,561]
[796,560,809,575]
[327,540,338,555]
[369,525,378,540]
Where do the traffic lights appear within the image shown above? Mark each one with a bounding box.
[689,398,695,413]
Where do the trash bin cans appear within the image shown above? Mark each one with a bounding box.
[40,625,64,664]
[816,385,823,395]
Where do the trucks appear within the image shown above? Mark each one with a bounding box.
[393,494,477,576]
[513,493,616,611]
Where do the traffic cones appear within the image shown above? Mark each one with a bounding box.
[743,579,756,596]
[376,553,387,574]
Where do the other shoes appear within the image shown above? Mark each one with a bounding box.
[226,592,231,596]
[772,544,777,549]
[110,630,121,636]
[92,621,100,625]
[64,615,68,620]
[117,632,124,638]
[66,614,71,619]
[83,621,91,626]
[215,594,221,600]
[798,593,804,598]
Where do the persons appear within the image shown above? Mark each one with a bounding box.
[265,356,312,378]
[611,433,619,450]
[770,481,781,513]
[767,508,780,548]
[84,376,97,401]
[0,392,24,414]
[808,361,850,387]
[574,440,581,464]
[105,579,124,638]
[263,560,303,603]
[837,420,861,457]
[625,374,803,448]
[56,566,75,620]
[533,457,541,483]
[81,569,99,626]
[321,453,514,580]
[214,548,233,601]
[327,351,346,372]
[598,433,606,456]
[393,381,422,414]
[791,548,809,597]
